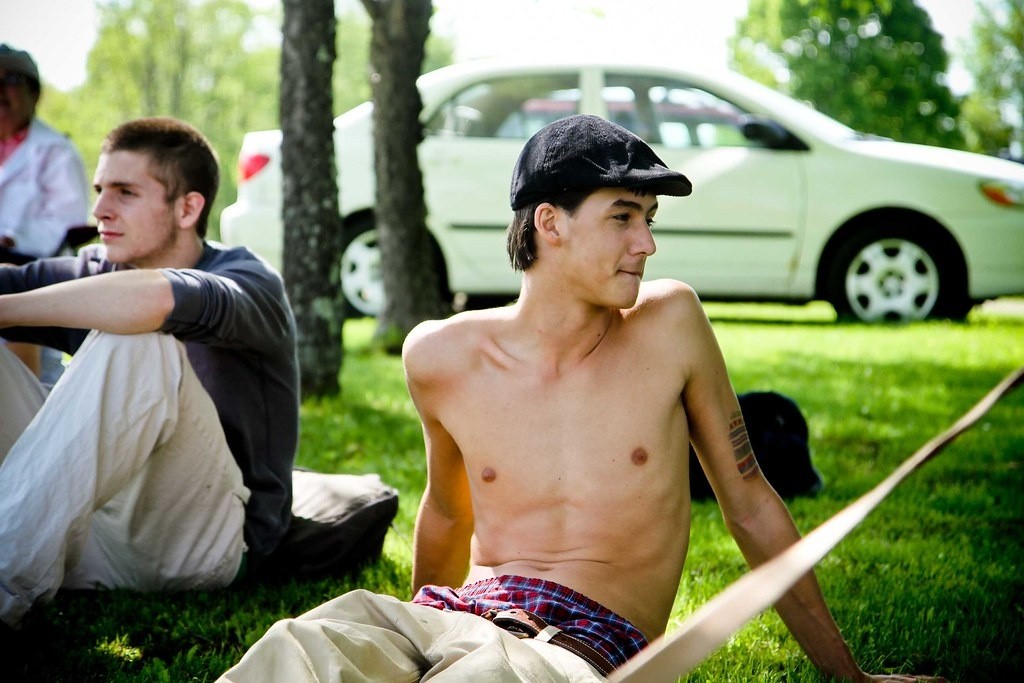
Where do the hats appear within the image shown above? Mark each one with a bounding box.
[509,114,693,211]
[0,43,40,84]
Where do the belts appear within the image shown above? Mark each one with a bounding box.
[492,608,619,679]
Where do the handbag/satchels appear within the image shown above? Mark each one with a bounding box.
[689,392,822,504]
[252,469,398,584]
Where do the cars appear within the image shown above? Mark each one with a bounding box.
[214,51,1023,329]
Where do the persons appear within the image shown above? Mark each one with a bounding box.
[214,115,945,683]
[0,42,299,637]
